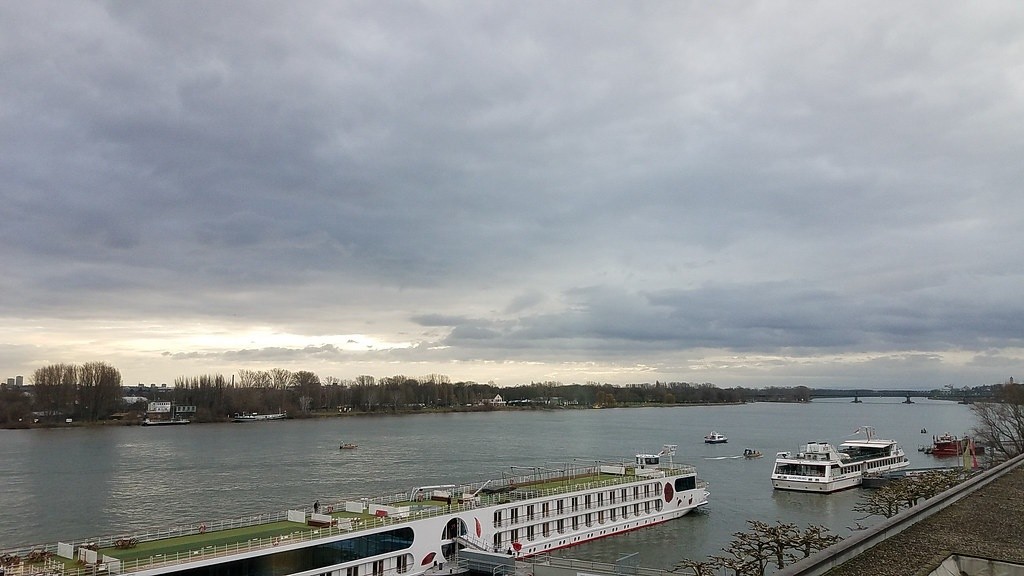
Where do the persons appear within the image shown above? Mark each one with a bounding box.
[314,500,319,514]
[508,547,512,555]
[447,495,451,513]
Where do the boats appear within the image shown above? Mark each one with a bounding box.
[745,453,764,459]
[704,435,728,443]
[0,443,710,576]
[342,444,358,449]
[771,427,910,494]
[932,431,962,458]
